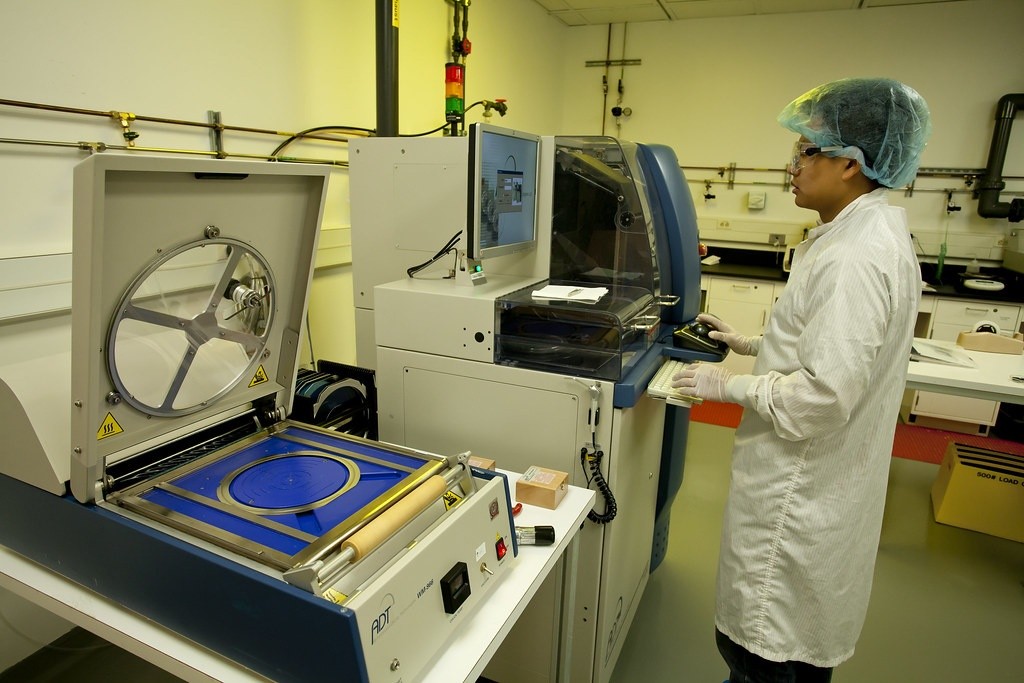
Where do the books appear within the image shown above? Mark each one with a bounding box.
[910,338,976,367]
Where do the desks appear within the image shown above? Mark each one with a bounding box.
[907,335,1024,404]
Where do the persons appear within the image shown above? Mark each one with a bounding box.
[671,78,930,683]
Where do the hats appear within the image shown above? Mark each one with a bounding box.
[777,77,932,189]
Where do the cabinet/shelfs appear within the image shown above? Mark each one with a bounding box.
[702,275,779,375]
[901,294,1023,436]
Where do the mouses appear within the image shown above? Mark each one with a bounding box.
[692,323,712,338]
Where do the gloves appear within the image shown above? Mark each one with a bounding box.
[672,364,734,403]
[695,313,754,356]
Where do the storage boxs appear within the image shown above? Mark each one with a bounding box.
[467,456,498,474]
[516,466,570,510]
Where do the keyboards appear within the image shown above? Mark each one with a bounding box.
[648,360,703,404]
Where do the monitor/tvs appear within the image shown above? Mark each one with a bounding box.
[466,121,541,259]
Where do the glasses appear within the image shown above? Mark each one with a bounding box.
[791,141,846,172]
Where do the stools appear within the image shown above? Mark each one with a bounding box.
[928,440,1024,542]
